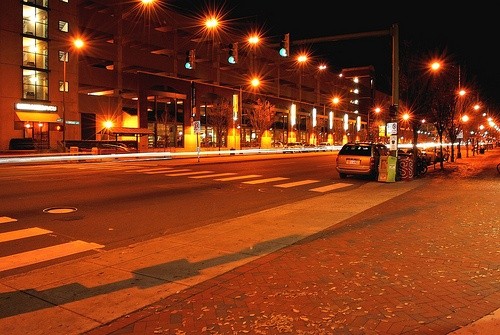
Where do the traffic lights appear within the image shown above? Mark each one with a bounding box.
[227,41,238,64]
[279,33,289,58]
[184,49,196,70]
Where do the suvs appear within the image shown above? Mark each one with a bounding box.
[335,140,390,179]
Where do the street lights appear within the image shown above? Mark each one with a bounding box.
[62,37,85,147]
[427,59,500,164]
[115,0,156,127]
[172,17,218,146]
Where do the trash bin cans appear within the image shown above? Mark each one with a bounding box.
[398,153,415,182]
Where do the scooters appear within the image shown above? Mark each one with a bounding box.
[397,148,429,176]
